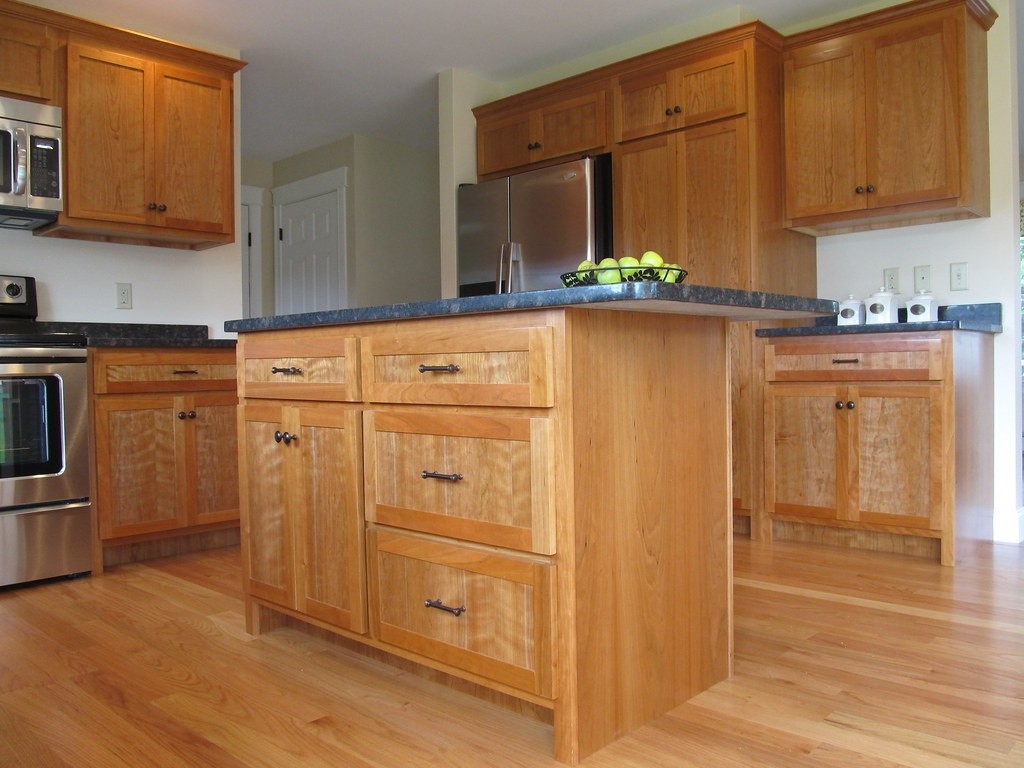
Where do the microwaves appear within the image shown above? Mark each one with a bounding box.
[0,97,64,230]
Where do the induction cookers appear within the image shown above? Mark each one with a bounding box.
[0,275,86,347]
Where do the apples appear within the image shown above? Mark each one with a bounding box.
[576,251,682,285]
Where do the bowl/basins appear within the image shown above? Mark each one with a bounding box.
[560,266,688,288]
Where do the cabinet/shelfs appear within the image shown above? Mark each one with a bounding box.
[476,79,611,182]
[0,14,59,106]
[781,0,998,236]
[236,324,369,644]
[760,330,994,568]
[32,32,236,251]
[360,309,565,726]
[86,346,241,578]
[611,38,817,535]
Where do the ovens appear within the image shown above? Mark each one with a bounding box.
[0,348,92,587]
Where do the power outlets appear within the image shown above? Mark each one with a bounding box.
[883,266,904,294]
[115,282,133,309]
[914,264,934,294]
[950,262,969,291]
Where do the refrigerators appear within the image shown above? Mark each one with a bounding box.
[457,157,613,298]
